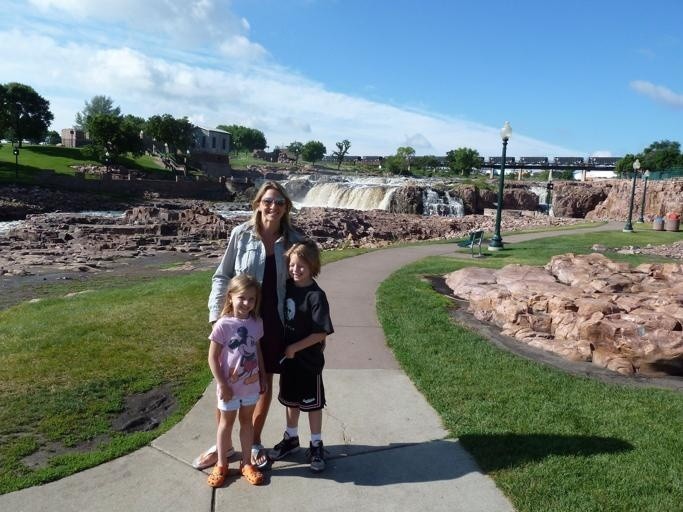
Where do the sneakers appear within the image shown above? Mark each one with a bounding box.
[268,431,299,460]
[310,441,326,474]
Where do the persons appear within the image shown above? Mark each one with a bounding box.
[206,273,269,490]
[192,178,306,469]
[267,237,334,473]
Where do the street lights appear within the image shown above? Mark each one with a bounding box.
[623,158,642,231]
[637,169,650,223]
[488,120,514,248]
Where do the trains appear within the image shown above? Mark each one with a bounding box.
[323,155,624,168]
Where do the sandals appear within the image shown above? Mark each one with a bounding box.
[208,462,229,488]
[240,460,265,485]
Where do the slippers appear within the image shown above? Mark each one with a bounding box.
[251,444,268,469]
[192,444,234,469]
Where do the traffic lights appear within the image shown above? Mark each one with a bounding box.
[13,150,19,155]
[547,184,553,190]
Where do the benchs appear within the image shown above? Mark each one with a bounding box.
[456,230,485,258]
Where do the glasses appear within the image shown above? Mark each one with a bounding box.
[262,198,286,206]
